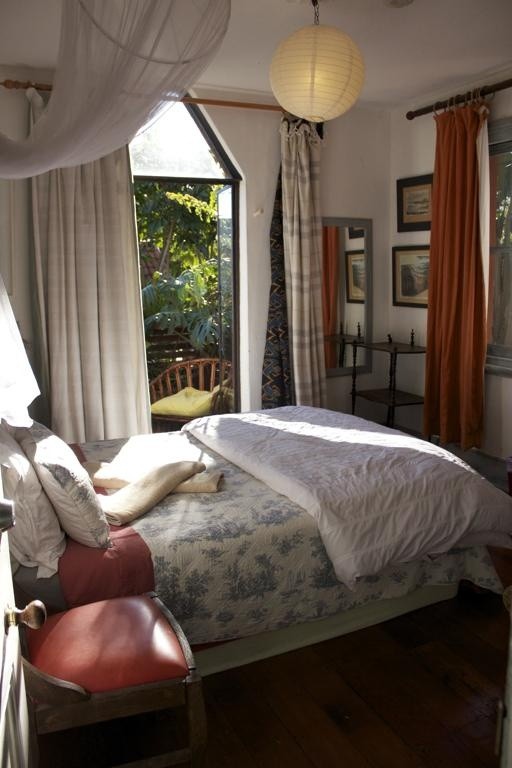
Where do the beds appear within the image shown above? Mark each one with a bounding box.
[13,404,512,758]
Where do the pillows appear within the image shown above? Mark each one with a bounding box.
[0,417,108,579]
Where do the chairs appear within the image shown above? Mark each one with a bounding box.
[18,590,208,767]
[148,358,232,431]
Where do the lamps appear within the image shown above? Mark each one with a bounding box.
[269,1,367,127]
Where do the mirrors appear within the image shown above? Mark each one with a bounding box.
[319,214,374,378]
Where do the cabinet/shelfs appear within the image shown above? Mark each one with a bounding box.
[352,341,426,431]
[324,333,364,367]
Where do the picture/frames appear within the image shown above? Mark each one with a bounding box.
[396,172,433,233]
[344,250,365,304]
[391,245,430,309]
